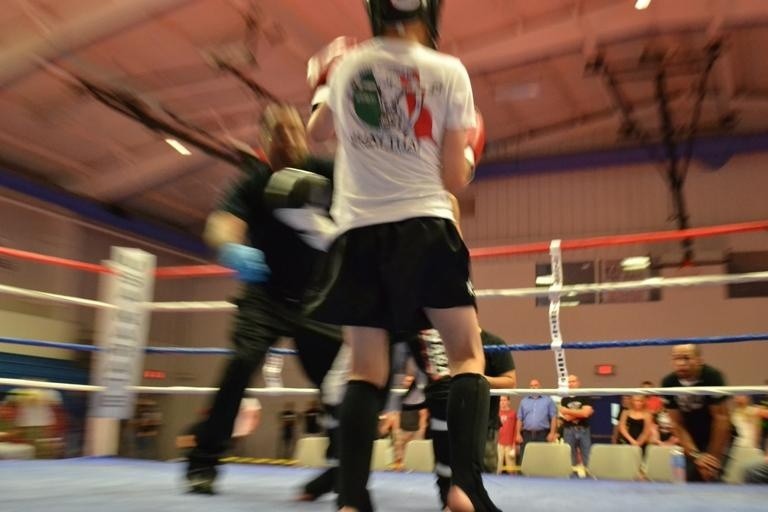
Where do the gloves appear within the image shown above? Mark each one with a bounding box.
[463,107,486,175]
[218,243,268,281]
[306,32,357,116]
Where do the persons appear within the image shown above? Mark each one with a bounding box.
[497,396,518,473]
[231,397,262,459]
[726,393,767,482]
[613,381,683,457]
[1,378,66,459]
[183,102,341,495]
[560,375,593,479]
[660,345,738,483]
[379,376,428,470]
[276,400,300,461]
[264,155,463,512]
[119,399,162,460]
[298,399,326,435]
[516,379,558,475]
[302,0,501,511]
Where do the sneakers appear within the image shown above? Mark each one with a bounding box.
[190,477,210,491]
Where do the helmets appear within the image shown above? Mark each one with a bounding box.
[361,0,440,38]
